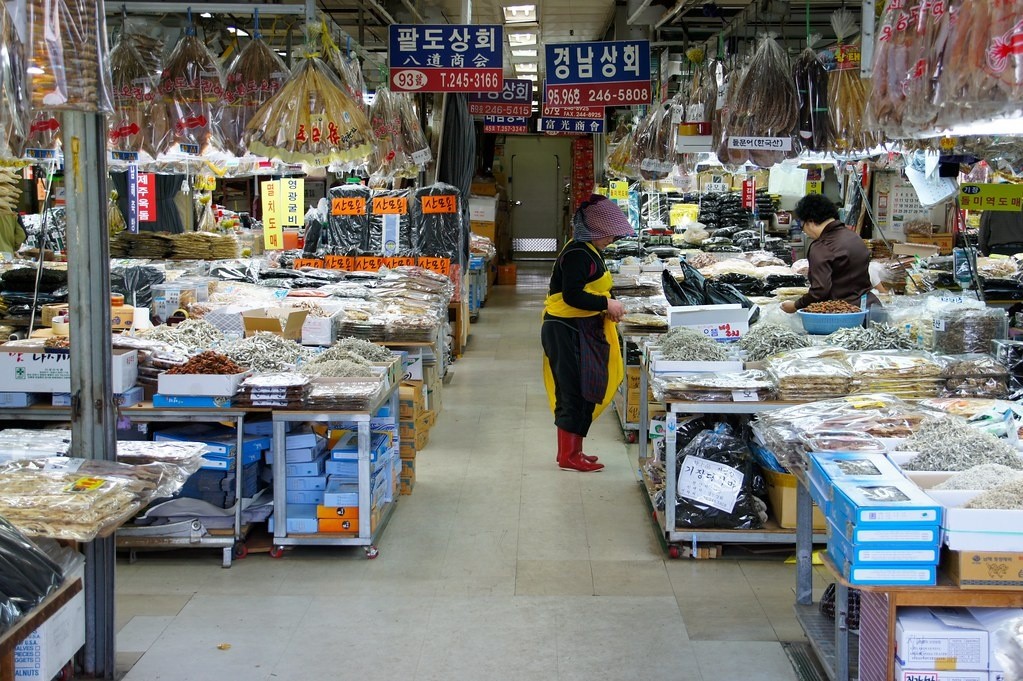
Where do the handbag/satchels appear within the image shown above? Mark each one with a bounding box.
[579,328,611,405]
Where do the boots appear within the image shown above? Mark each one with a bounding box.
[557,426,598,463]
[559,427,605,473]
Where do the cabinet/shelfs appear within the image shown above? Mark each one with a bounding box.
[5,380,401,568]
[638,356,828,557]
[796,477,1022,681]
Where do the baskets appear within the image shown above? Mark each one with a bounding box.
[797,308,869,334]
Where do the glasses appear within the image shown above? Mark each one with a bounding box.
[800,221,806,235]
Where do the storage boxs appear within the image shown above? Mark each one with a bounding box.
[761,466,828,529]
[805,437,1023,681]
[241,305,345,347]
[14,587,85,681]
[906,233,953,256]
[615,365,666,423]
[153,389,403,532]
[0,340,144,407]
[391,347,435,495]
[666,303,758,343]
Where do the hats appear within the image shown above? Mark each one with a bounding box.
[572,194,638,241]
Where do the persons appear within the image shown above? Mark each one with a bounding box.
[539,193,637,474]
[780,193,886,329]
